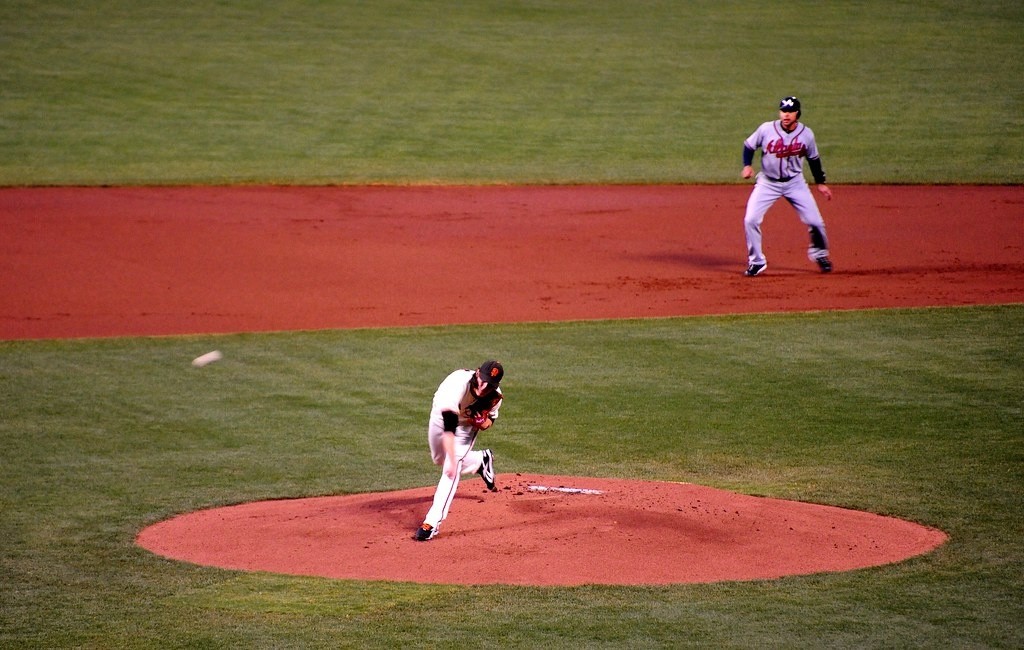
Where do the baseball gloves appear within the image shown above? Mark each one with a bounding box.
[462,390,504,432]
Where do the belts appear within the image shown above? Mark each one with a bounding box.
[772,178,792,182]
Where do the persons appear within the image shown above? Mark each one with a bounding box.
[412,362,503,543]
[742,96,834,277]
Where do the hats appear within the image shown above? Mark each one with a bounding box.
[778,97,801,111]
[480,361,504,384]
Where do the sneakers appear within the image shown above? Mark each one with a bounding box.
[817,257,832,272]
[416,524,440,541]
[746,264,767,276]
[476,448,494,491]
